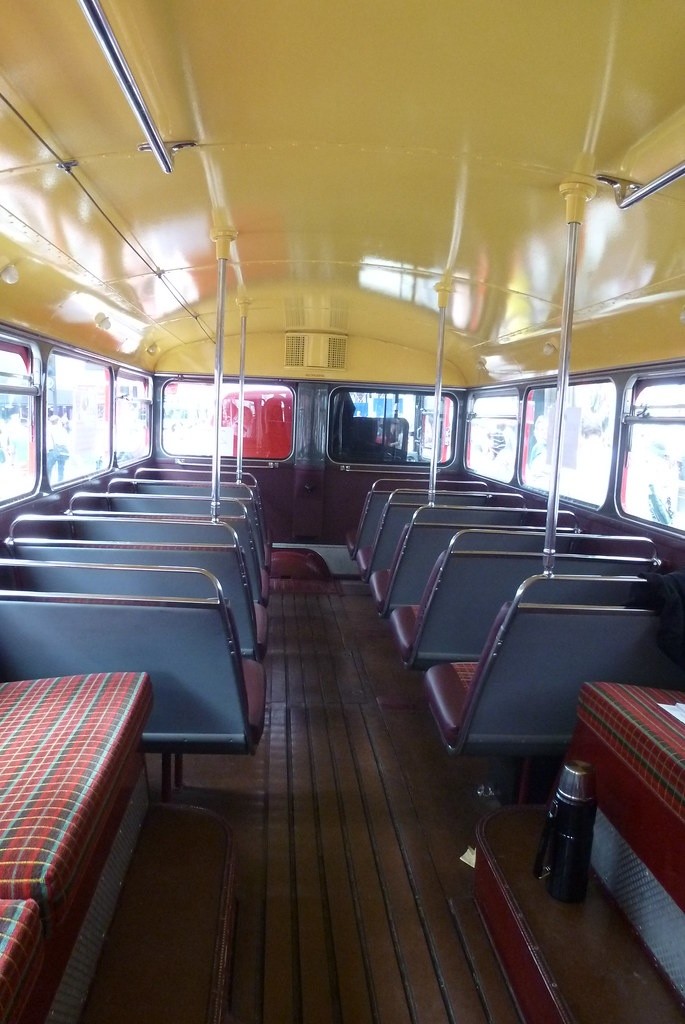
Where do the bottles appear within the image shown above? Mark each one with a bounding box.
[534,759,598,904]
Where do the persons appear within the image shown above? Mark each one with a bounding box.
[233,406,253,439]
[45,414,68,482]
[529,415,548,463]
[493,423,517,458]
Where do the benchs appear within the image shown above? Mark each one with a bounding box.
[345,478,685,1009]
[0,467,272,1024]
